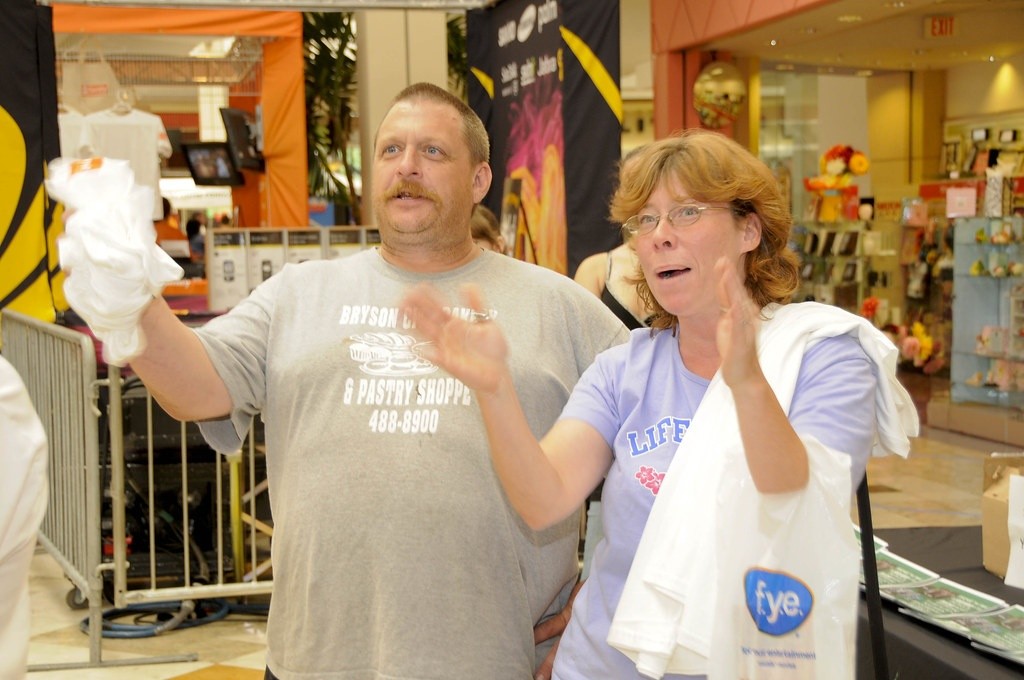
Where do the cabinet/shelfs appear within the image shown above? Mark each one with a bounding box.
[799,216,1024,411]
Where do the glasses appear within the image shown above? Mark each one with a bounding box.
[621,204,747,236]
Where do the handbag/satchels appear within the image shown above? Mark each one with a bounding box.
[706,433,859,680]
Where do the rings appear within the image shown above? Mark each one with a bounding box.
[476,312,490,321]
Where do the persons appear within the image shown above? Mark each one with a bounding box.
[125,81,631,680]
[1,356,49,680]
[401,131,921,680]
[574,241,662,332]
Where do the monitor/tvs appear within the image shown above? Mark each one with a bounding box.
[180,108,265,185]
